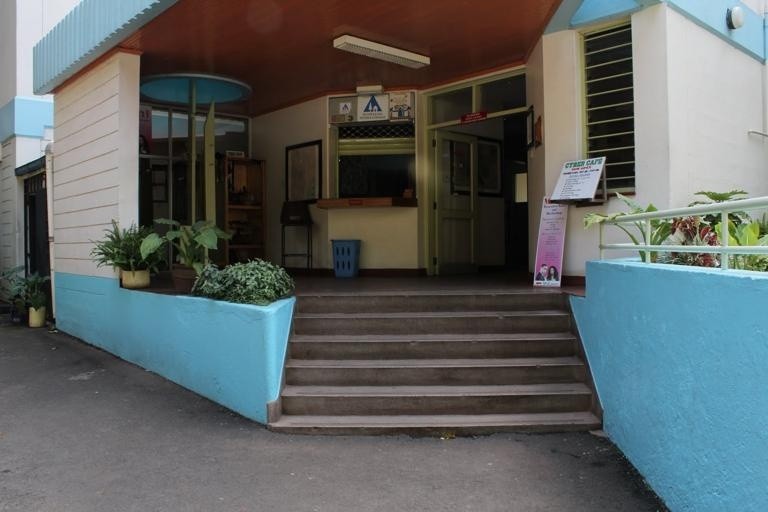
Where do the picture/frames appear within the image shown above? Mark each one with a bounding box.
[524,103,535,150]
[284,138,323,204]
[450,139,504,198]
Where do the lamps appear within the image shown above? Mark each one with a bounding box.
[332,33,430,71]
[356,85,384,95]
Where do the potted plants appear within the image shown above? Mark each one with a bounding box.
[87,212,169,291]
[0,262,32,327]
[151,215,232,291]
[16,267,54,329]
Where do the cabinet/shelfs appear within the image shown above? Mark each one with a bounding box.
[216,155,269,270]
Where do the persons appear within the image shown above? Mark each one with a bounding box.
[545,265,559,281]
[534,263,547,281]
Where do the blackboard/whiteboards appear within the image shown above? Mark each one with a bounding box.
[548,156,606,203]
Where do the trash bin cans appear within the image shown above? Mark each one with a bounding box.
[330,240,361,279]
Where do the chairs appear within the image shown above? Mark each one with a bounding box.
[278,199,316,276]
